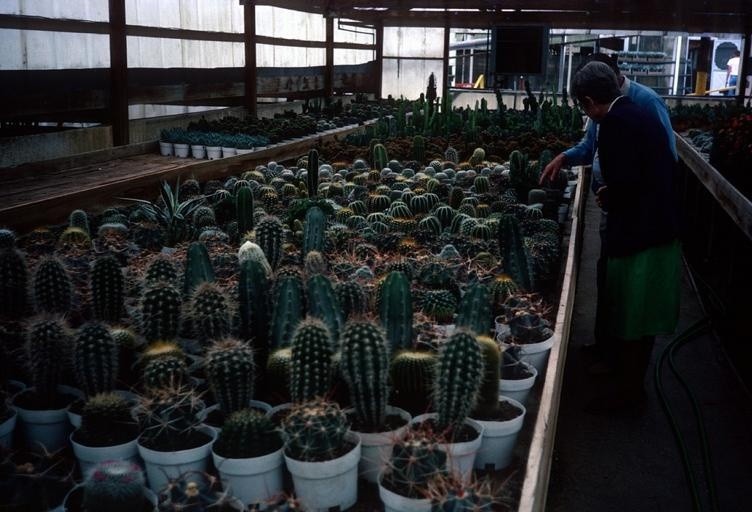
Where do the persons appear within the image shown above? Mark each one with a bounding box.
[726,51,744,96]
[570,60,680,420]
[538,57,678,381]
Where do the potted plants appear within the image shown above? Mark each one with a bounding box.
[666,103,713,132]
[615,51,667,76]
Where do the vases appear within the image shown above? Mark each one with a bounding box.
[711,145,752,201]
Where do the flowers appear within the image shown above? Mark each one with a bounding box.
[710,101,752,161]
